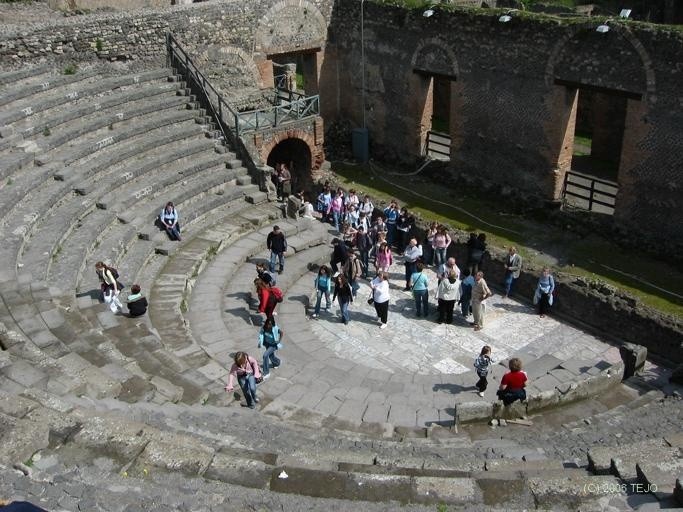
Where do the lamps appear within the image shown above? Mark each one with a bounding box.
[616,8,632,21]
[596,19,615,33]
[423,4,437,19]
[499,9,518,23]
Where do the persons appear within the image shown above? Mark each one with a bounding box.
[473,345,494,398]
[160,201,180,235]
[254,278,277,326]
[532,267,555,318]
[267,225,287,274]
[127,284,148,316]
[256,263,276,286]
[95,261,121,303]
[496,357,527,407]
[257,317,284,379]
[223,351,260,409]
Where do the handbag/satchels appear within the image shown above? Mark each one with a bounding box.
[269,286,284,303]
[255,370,264,384]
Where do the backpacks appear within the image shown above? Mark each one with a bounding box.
[104,267,120,281]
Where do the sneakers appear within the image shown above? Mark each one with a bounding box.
[478,390,485,397]
[378,323,388,329]
[311,294,357,326]
